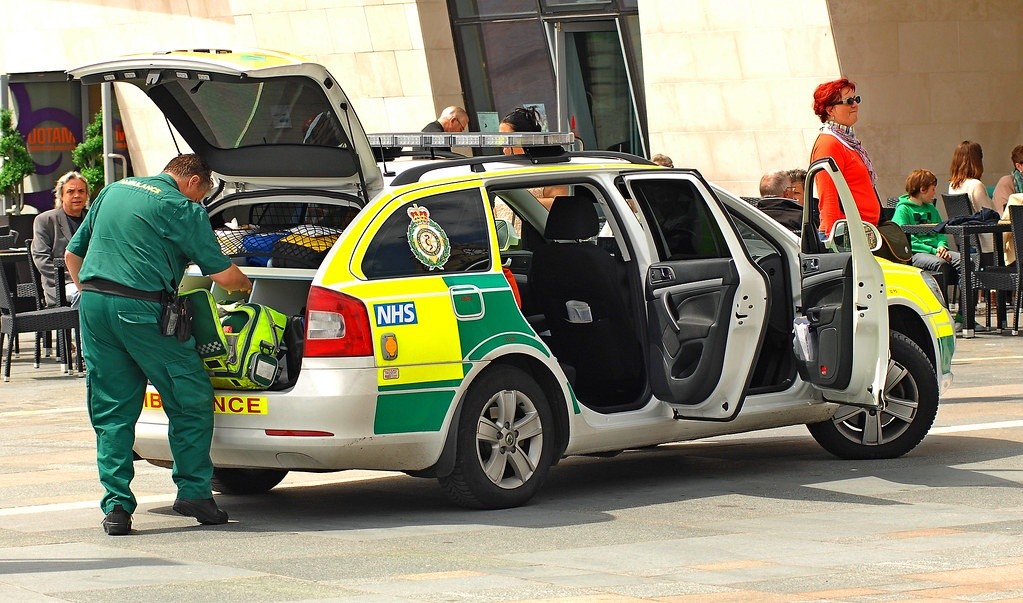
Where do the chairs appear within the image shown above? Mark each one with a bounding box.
[527,195,635,387]
[882,192,1023,338]
[0,215,79,383]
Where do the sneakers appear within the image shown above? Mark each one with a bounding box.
[953,314,985,332]
[102,503,133,535]
[173,496,229,526]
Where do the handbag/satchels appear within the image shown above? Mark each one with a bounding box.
[177,287,287,390]
[875,221,913,264]
[284,305,306,384]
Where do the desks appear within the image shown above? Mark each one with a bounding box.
[901,223,1012,337]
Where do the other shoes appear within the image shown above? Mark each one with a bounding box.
[1005,301,1022,312]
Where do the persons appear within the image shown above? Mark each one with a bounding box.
[31,170,90,310]
[993,145,1023,220]
[810,78,882,242]
[413,106,470,160]
[752,169,826,255]
[498,106,542,155]
[948,140,996,254]
[890,169,984,332]
[64,153,253,536]
[650,153,698,235]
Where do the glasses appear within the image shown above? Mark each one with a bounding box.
[830,95,862,106]
[452,115,464,132]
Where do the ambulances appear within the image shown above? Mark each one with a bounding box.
[63,50,957,511]
